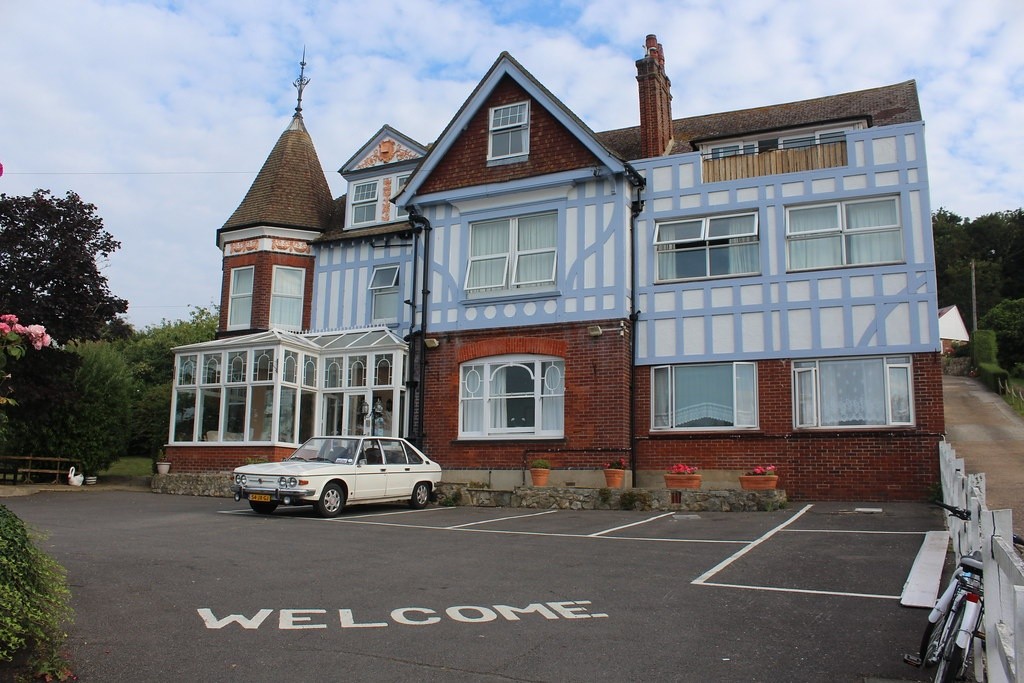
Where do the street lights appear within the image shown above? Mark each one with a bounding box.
[361,398,383,448]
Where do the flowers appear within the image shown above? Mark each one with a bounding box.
[603,458,626,470]
[665,463,698,475]
[745,466,776,476]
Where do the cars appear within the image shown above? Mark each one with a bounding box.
[231,435,443,518]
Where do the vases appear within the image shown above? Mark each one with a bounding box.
[663,474,701,488]
[738,476,778,490]
[603,469,624,488]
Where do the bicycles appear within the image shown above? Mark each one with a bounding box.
[904,500,1024,683]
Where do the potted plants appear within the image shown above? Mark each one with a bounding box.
[81,459,97,484]
[156,448,172,474]
[530,459,549,486]
